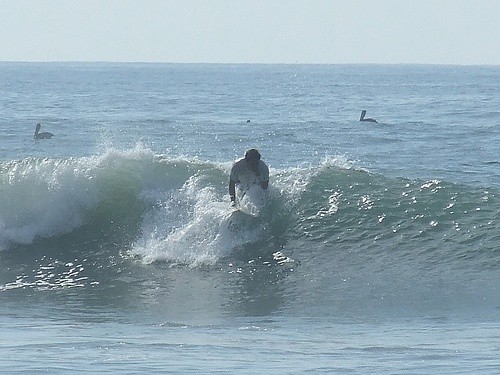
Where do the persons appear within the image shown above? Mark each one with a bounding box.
[226,149,270,207]
